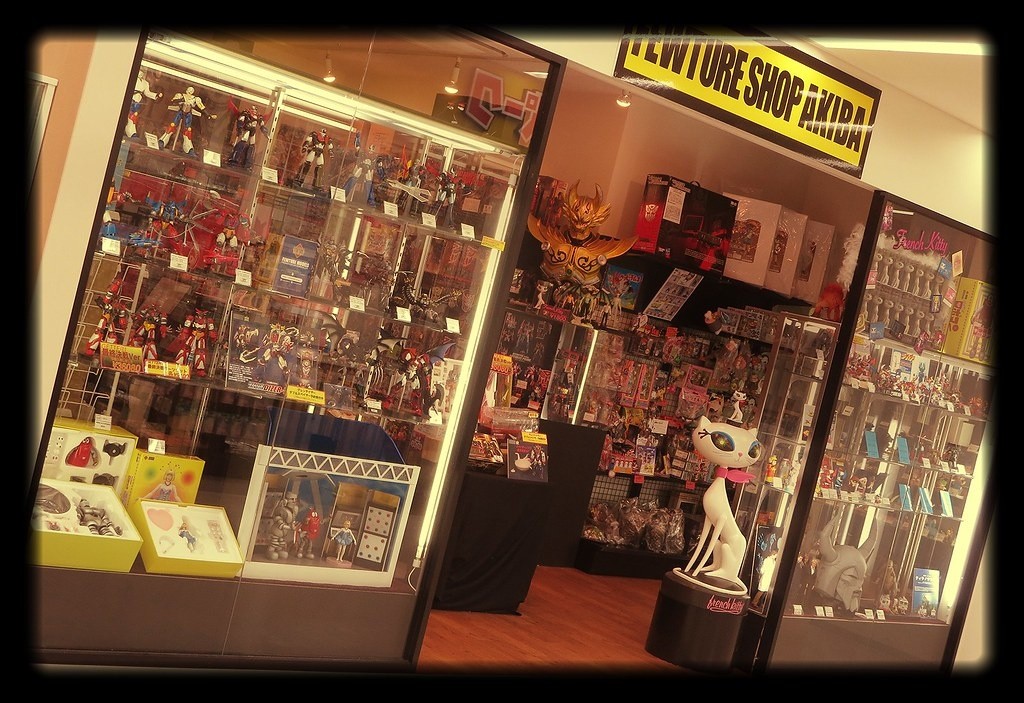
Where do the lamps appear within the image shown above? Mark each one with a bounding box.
[141,59,359,135]
[411,172,521,570]
[616,89,634,109]
[445,57,464,95]
[322,49,337,84]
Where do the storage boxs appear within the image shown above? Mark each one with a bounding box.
[29,416,245,581]
[410,421,448,463]
[258,230,320,301]
[940,273,1000,371]
[619,357,715,411]
[475,353,540,435]
[636,177,838,312]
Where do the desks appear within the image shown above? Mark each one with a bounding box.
[532,417,611,568]
[392,438,554,615]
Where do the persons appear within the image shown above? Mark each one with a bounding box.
[750,549,818,612]
[178,521,198,552]
[329,520,357,563]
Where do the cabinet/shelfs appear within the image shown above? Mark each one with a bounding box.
[733,311,1000,672]
[67,65,525,458]
